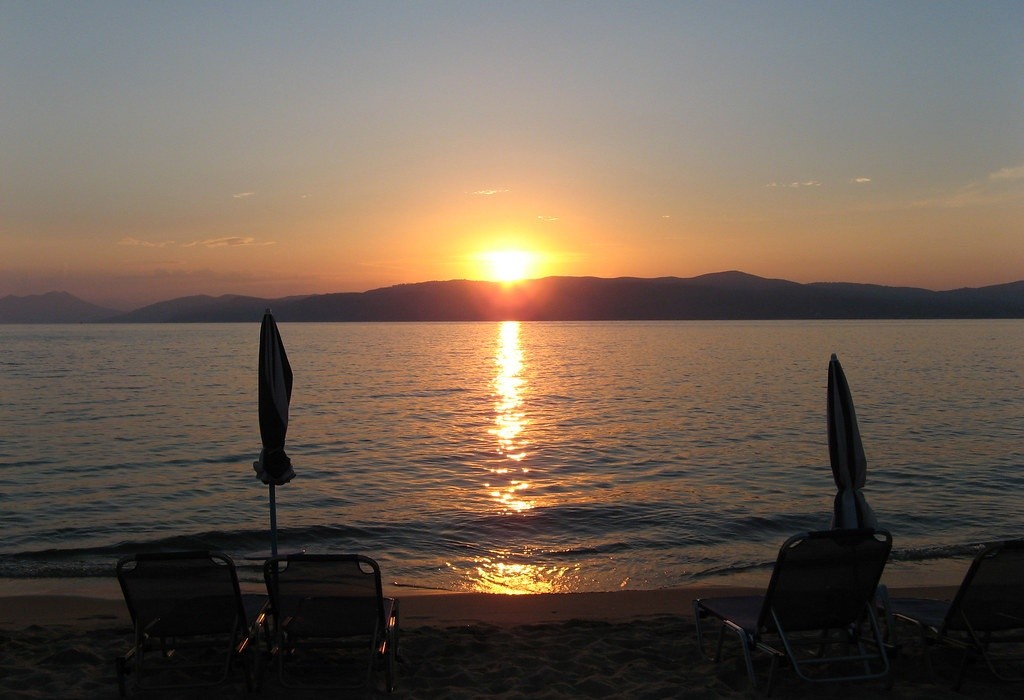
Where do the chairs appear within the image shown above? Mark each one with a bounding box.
[265,553,397,696]
[892,537,1024,682]
[692,528,892,692]
[113,551,272,700]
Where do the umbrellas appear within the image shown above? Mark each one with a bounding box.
[253,308,296,635]
[826,352,878,531]
